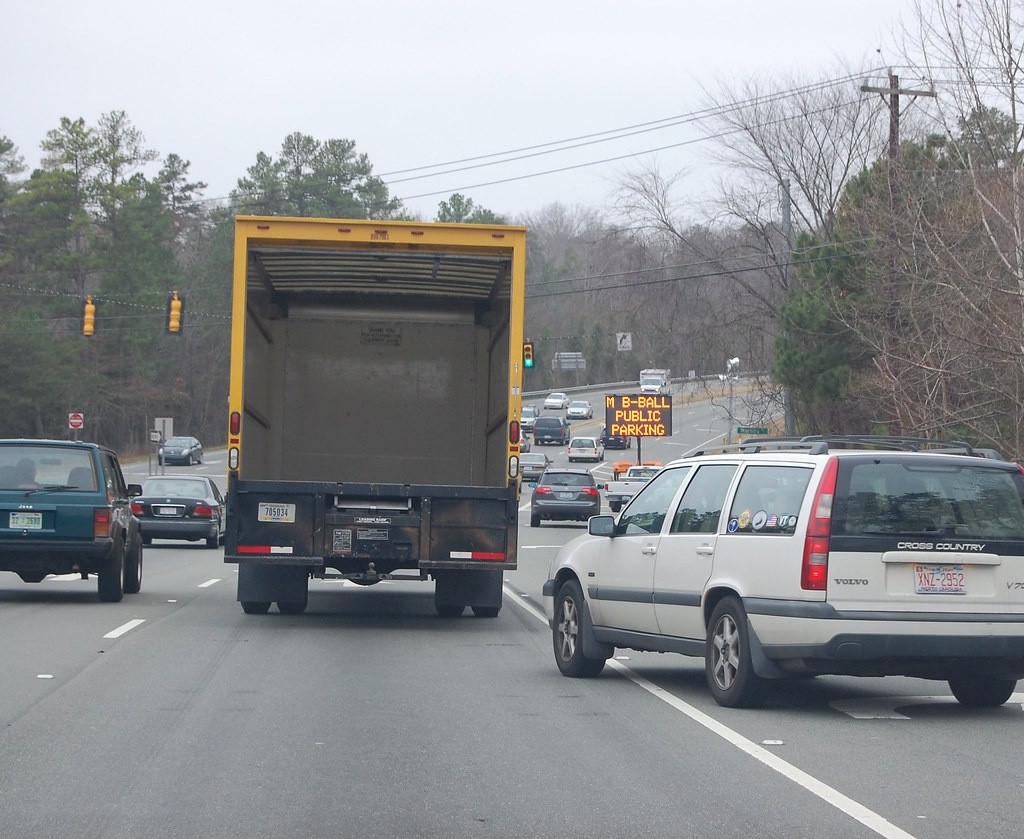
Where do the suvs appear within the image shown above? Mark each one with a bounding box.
[529,466,605,528]
[0,437,143,603]
[568,435,605,462]
[532,417,571,446]
[542,435,1024,710]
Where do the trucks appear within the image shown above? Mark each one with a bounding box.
[636,368,671,395]
[223,215,526,619]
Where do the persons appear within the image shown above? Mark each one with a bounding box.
[17,459,36,484]
[649,486,709,533]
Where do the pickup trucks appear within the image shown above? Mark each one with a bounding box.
[604,466,663,513]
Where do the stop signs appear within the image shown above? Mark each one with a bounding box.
[69,412,84,430]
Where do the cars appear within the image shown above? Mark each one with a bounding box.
[600,428,631,450]
[519,407,537,432]
[519,428,531,453]
[132,474,225,550]
[520,453,554,481]
[566,401,594,421]
[158,436,203,466]
[527,404,542,420]
[543,393,569,410]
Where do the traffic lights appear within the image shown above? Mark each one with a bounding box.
[523,341,534,369]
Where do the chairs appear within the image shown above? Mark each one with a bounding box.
[67,467,95,491]
[577,477,587,486]
[0,466,26,490]
[156,485,165,495]
